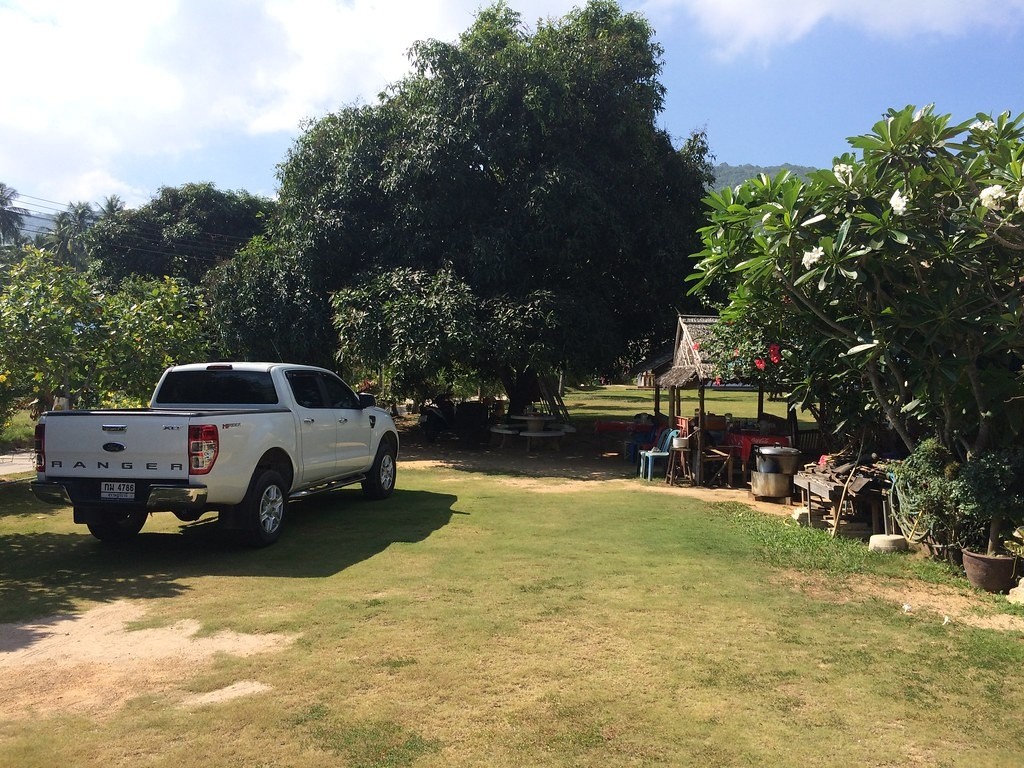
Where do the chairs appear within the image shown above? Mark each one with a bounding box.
[627,422,680,482]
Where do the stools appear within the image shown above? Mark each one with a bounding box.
[666,448,693,486]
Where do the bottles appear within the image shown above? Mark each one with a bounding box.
[708,413,716,417]
[695,409,699,426]
[725,413,732,424]
[768,415,774,422]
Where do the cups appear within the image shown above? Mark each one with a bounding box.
[733,421,740,431]
[784,422,791,435]
[727,422,733,431]
[769,423,776,433]
[640,413,648,424]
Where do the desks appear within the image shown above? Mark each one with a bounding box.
[594,421,653,457]
[725,431,789,487]
[511,414,556,431]
[793,473,883,535]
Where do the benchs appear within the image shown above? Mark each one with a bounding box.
[490,422,576,452]
[702,439,734,486]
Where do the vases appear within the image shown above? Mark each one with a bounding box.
[957,447,1024,595]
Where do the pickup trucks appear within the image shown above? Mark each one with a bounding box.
[30,361,401,550]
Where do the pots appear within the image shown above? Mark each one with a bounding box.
[672,437,688,448]
[753,442,802,474]
